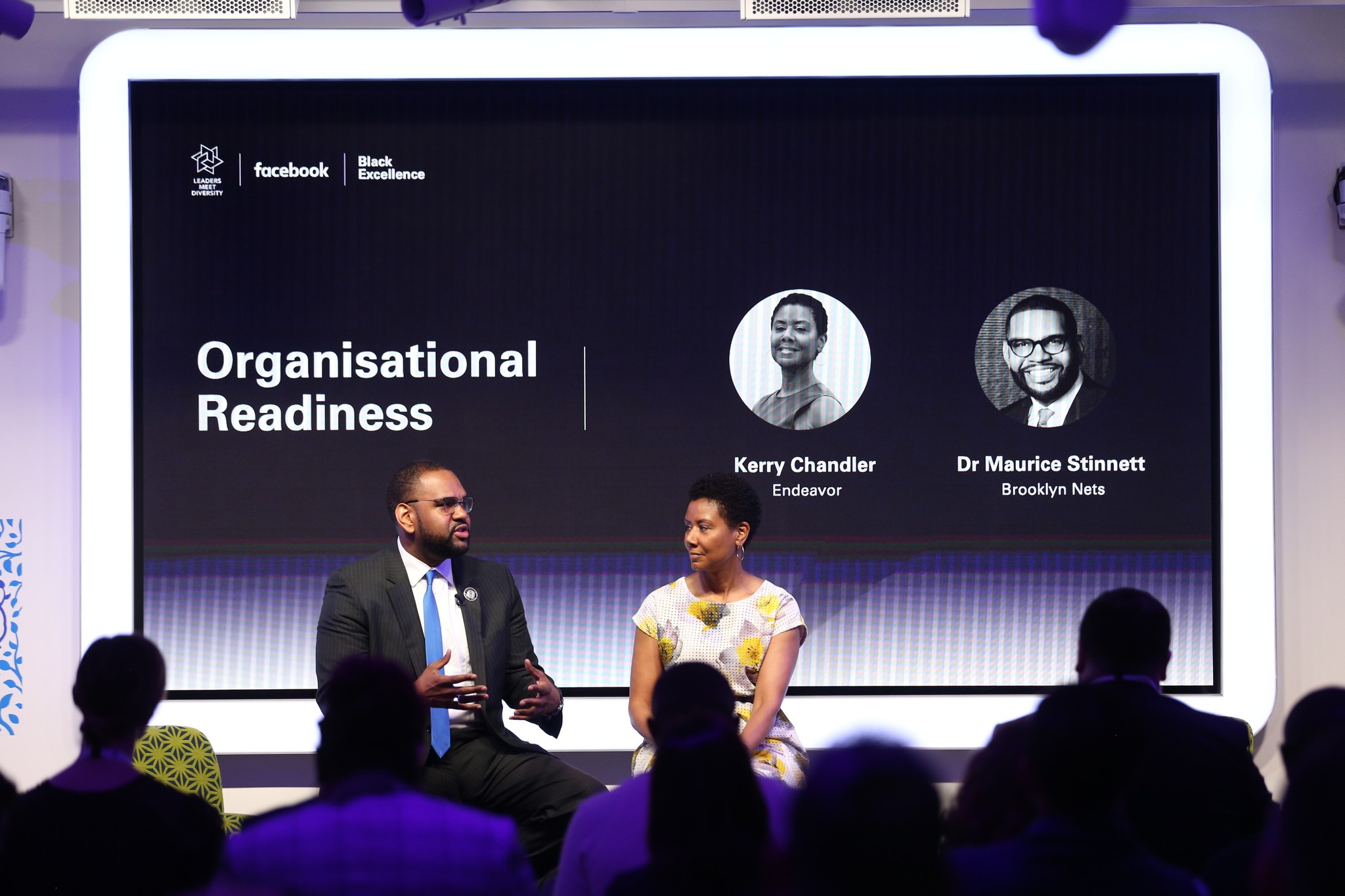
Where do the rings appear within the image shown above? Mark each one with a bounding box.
[453,694,460,706]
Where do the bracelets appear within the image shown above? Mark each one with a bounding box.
[552,699,564,717]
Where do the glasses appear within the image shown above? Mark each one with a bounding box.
[1006,334,1071,358]
[405,497,475,513]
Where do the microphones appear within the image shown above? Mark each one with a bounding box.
[455,593,465,605]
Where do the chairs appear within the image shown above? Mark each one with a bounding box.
[126,724,255,838]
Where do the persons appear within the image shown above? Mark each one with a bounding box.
[19,636,227,895]
[627,473,811,795]
[552,662,1212,896]
[223,654,536,896]
[1248,688,1345,896]
[996,292,1110,429]
[752,292,845,430]
[941,585,1278,896]
[314,459,613,877]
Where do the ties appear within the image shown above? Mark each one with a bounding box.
[424,570,451,759]
